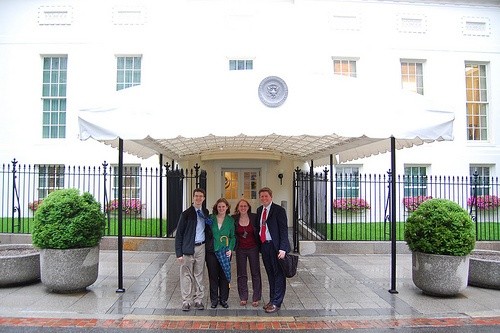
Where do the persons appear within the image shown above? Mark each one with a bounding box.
[205,198,236,308]
[253,187,291,314]
[232,199,262,307]
[174,188,210,311]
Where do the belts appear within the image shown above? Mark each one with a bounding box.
[194,241,205,246]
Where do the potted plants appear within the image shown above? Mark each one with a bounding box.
[403,197,476,298]
[32,188,106,292]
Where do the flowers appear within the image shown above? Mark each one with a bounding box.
[333,198,370,209]
[28,200,42,209]
[467,195,500,210]
[106,199,141,217]
[403,196,432,210]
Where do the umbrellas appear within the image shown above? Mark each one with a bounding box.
[276,251,298,278]
[215,235,233,289]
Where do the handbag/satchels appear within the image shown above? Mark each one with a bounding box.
[276,251,298,278]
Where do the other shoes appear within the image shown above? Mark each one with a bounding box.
[181,303,191,311]
[252,300,259,307]
[239,300,247,306]
[194,302,204,310]
[266,304,280,313]
[211,305,217,308]
[263,302,272,309]
[220,302,229,308]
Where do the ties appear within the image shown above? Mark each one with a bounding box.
[260,207,267,244]
[198,210,211,225]
